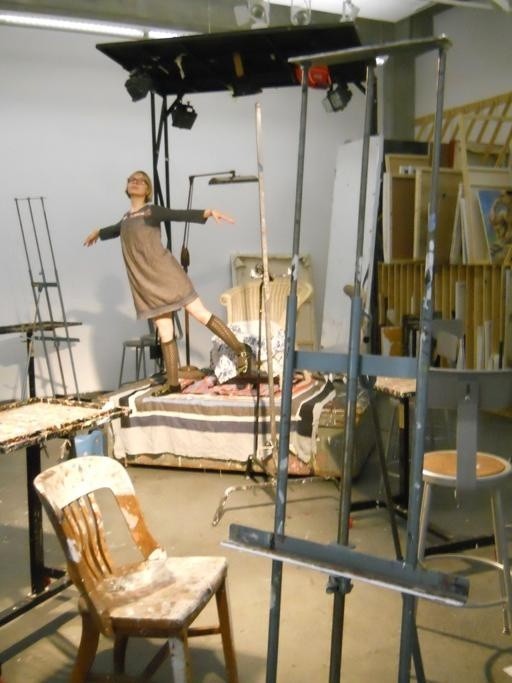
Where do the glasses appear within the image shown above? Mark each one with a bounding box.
[128,176,150,186]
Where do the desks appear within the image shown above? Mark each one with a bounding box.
[0,394,137,632]
[1,318,82,398]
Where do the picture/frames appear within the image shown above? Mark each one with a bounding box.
[381,138,512,267]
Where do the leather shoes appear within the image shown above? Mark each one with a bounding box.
[150,381,183,397]
[235,342,255,379]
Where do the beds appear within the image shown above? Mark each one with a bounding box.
[94,365,373,482]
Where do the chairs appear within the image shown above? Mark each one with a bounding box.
[219,272,314,331]
[32,451,238,683]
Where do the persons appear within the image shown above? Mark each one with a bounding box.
[83,171,252,395]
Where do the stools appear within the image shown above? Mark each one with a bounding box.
[118,337,146,385]
[136,332,165,377]
[418,448,512,636]
[352,362,498,555]
